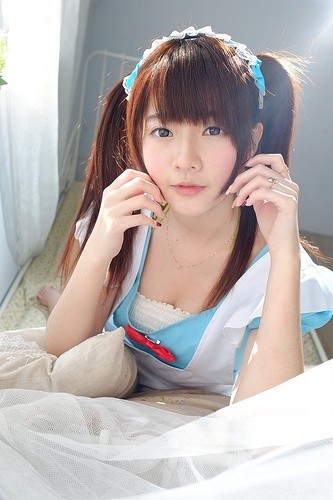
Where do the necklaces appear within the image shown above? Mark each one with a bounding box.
[162,212,240,269]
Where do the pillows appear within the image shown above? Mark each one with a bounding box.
[1,326,136,400]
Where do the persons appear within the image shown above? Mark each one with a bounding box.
[30,26,333,408]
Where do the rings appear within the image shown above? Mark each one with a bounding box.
[267,177,276,190]
[278,166,289,176]
